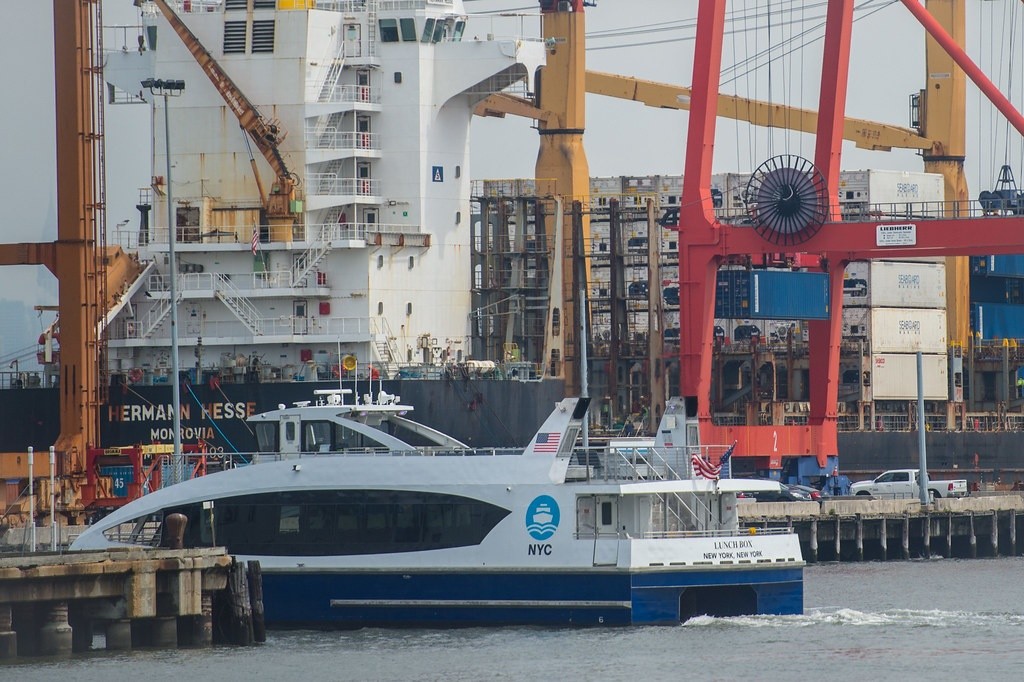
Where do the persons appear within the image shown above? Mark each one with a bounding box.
[625,421,634,437]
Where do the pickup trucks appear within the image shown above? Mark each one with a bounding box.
[849,468,968,499]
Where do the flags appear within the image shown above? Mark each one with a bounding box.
[692,440,737,480]
[534,432,561,452]
[252,217,260,256]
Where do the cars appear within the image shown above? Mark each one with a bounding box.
[786,483,822,510]
[739,478,810,502]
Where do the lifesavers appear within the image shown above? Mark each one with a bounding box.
[128,322,134,335]
[343,355,356,371]
[334,364,345,377]
[128,368,143,383]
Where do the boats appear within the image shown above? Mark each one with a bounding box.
[61,388,804,629]
